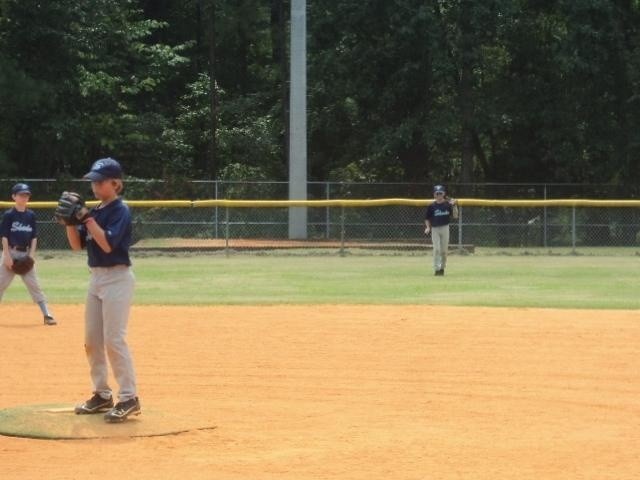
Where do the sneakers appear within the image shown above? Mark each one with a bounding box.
[435,269,444,276]
[44,314,56,325]
[74,392,114,414]
[104,397,141,423]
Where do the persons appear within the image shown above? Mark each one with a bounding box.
[424,184,459,277]
[0,183,57,326]
[53,156,142,424]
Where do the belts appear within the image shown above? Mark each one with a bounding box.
[11,245,27,252]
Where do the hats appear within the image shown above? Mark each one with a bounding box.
[83,158,121,181]
[11,183,31,194]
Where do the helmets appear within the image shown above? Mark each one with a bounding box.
[433,185,445,197]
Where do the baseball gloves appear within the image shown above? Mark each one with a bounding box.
[55,191,87,224]
[12,256,34,274]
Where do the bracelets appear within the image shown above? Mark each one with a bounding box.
[453,204,455,206]
